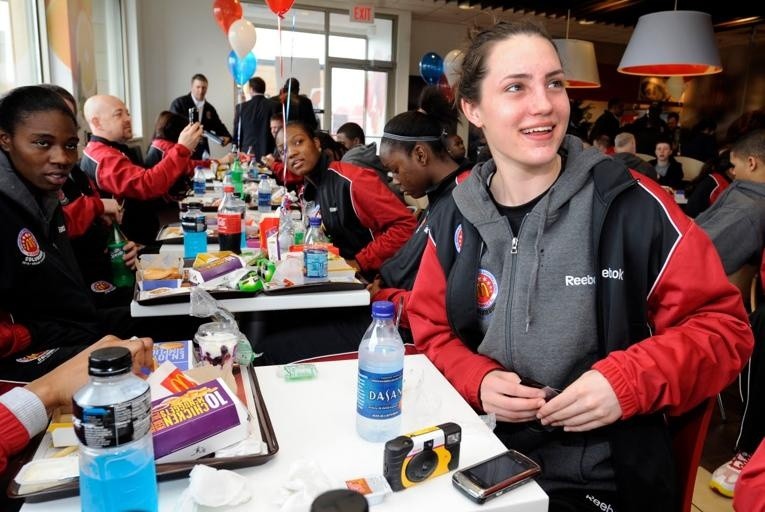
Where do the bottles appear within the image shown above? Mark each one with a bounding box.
[216,186,242,255]
[107,214,135,289]
[355,300,405,444]
[70,346,159,512]
[302,216,329,284]
[247,146,255,165]
[232,192,248,249]
[231,143,237,153]
[231,158,244,200]
[222,161,234,182]
[248,157,258,179]
[202,150,210,161]
[193,165,207,198]
[181,203,208,258]
[257,175,272,214]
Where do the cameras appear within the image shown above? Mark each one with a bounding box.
[382,422,462,492]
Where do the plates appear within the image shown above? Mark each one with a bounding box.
[249,203,301,215]
[178,200,218,215]
[154,221,221,246]
[262,276,366,296]
[6,345,279,504]
[134,289,261,307]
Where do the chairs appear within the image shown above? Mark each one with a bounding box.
[636,152,656,162]
[662,392,718,512]
[672,155,706,197]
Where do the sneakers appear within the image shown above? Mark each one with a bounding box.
[711,451,753,498]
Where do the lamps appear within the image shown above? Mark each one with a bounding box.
[553,8,601,86]
[617,0,724,76]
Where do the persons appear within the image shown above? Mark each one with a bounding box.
[170,73,232,160]
[0,334,153,473]
[144,110,235,196]
[666,111,685,142]
[282,78,318,133]
[335,123,365,152]
[692,109,765,276]
[679,116,720,172]
[79,94,204,254]
[256,88,473,364]
[442,133,476,165]
[270,111,283,139]
[274,120,421,273]
[592,96,625,149]
[407,18,756,510]
[311,131,393,188]
[609,132,657,183]
[0,85,189,381]
[647,142,684,181]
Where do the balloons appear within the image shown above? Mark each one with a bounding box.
[226,50,257,89]
[212,0,243,38]
[227,19,256,62]
[265,0,294,18]
[442,50,465,88]
[419,52,442,87]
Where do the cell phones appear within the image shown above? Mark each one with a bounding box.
[452,449,541,505]
[191,108,199,126]
